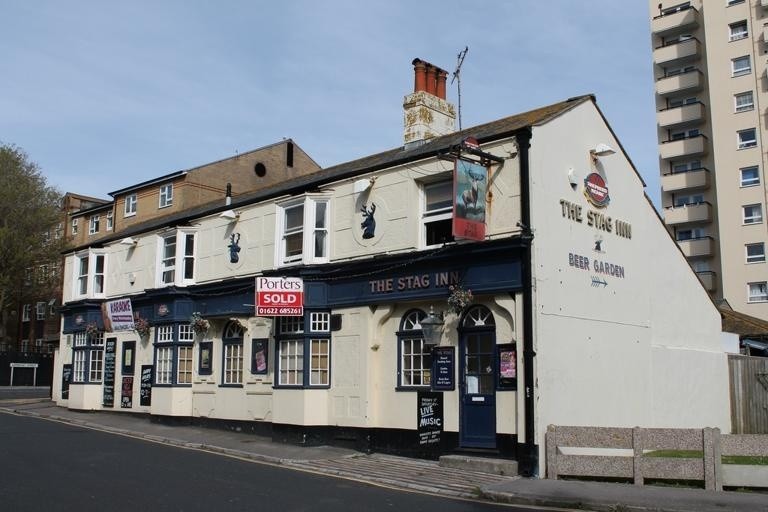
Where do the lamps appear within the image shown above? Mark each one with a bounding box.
[120,236,141,245]
[589,142,617,164]
[219,209,242,222]
[350,174,379,192]
[418,304,446,347]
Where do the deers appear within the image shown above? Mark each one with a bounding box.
[461,169,484,217]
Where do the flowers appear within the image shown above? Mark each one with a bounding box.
[157,303,169,315]
[133,318,151,340]
[190,311,210,336]
[85,320,100,335]
[448,284,474,313]
